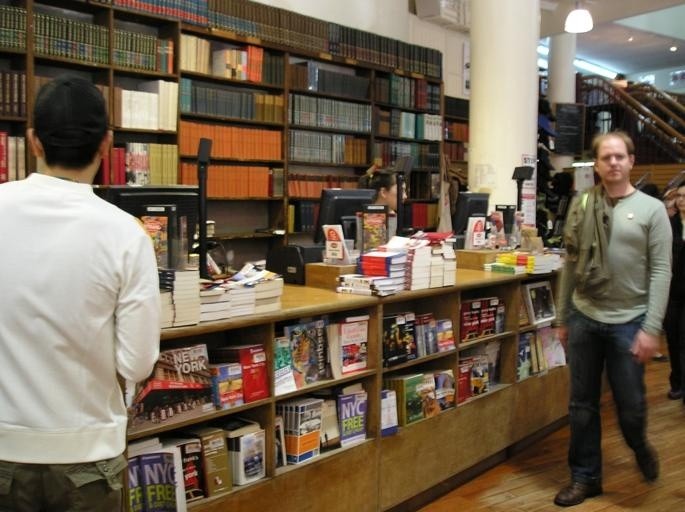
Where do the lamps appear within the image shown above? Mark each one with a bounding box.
[563,0,595,35]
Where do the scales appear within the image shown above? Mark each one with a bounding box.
[512,167,535,212]
[121,189,199,270]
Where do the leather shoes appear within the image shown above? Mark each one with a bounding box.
[552,479,606,507]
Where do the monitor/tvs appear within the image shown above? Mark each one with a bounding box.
[314,187,379,246]
[453,191,489,237]
[91,184,201,278]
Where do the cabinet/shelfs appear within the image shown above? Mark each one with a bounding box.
[0,0,446,287]
[124,253,572,511]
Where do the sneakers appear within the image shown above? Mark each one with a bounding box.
[632,443,660,483]
[668,389,685,400]
[652,351,668,362]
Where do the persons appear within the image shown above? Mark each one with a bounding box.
[649,183,676,365]
[552,127,676,507]
[666,175,685,402]
[0,72,164,512]
